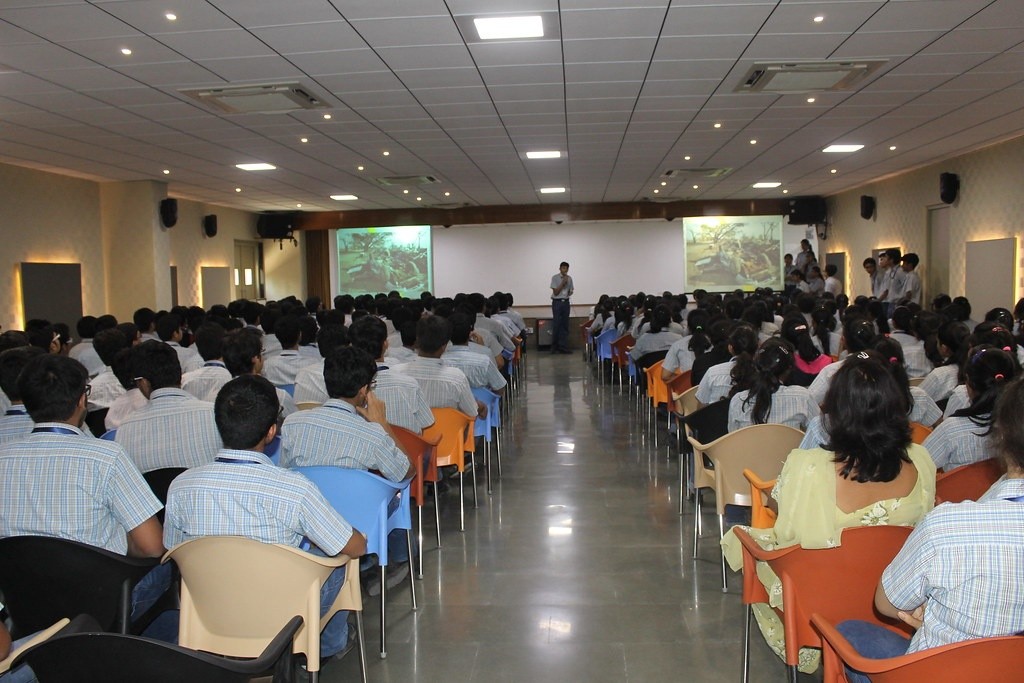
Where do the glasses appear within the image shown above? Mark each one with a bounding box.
[133,376,143,388]
[52,334,60,342]
[260,347,265,355]
[370,378,377,387]
[277,405,284,418]
[84,385,92,396]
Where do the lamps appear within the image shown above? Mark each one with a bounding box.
[203,214,218,237]
[160,198,178,229]
[860,195,875,219]
[939,172,959,203]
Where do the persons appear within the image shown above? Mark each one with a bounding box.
[0,291,525,683]
[550,262,574,351]
[580,235,1024,683]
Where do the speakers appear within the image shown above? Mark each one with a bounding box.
[939,171,961,205]
[204,212,219,236]
[536,317,554,352]
[859,193,876,221]
[159,196,178,230]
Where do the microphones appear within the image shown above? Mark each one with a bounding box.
[564,272,568,284]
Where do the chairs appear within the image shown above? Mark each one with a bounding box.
[0,318,527,683]
[580,299,1024,683]
